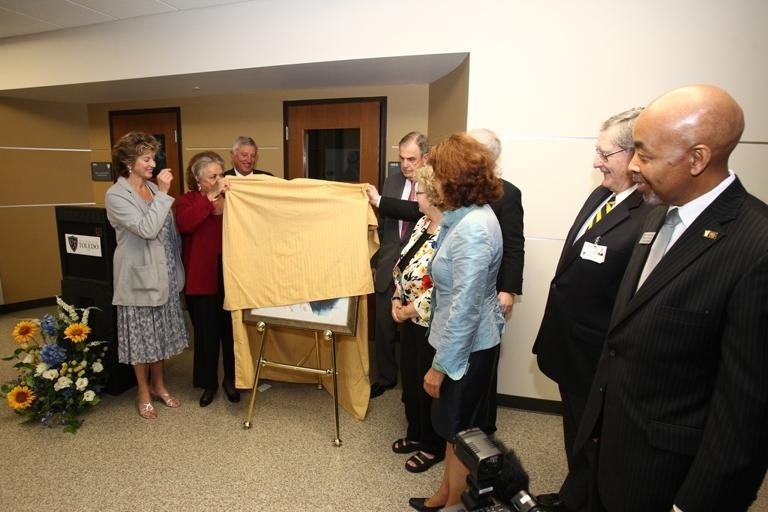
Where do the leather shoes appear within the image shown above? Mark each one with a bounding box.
[536,494,562,512]
[370,383,395,398]
[222,380,240,403]
[409,498,445,512]
[200,388,218,407]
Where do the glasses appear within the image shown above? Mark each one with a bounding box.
[601,149,625,163]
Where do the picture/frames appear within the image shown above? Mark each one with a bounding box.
[242,292,360,337]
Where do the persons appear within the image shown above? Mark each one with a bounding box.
[105,132,187,420]
[223,137,273,176]
[532,104,664,510]
[172,157,240,408]
[559,82,768,511]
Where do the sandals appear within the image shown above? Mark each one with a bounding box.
[136,392,157,419]
[150,384,181,408]
[405,451,446,473]
[392,438,424,453]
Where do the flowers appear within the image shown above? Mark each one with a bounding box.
[1,295,114,436]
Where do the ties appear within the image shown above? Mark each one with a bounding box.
[633,208,681,296]
[585,197,615,232]
[401,181,417,239]
